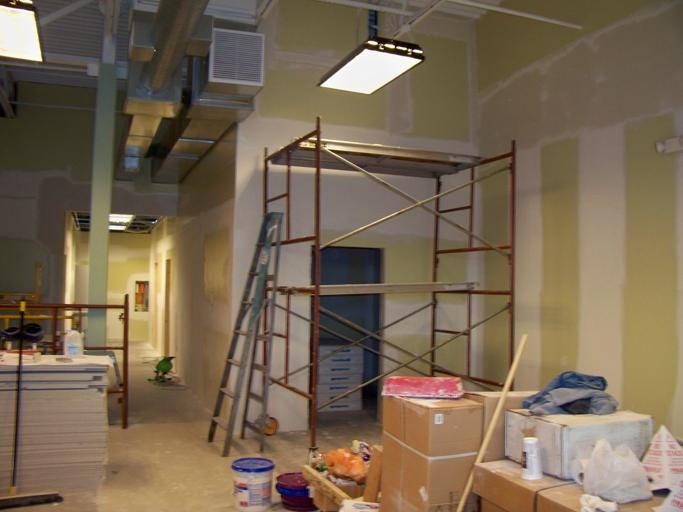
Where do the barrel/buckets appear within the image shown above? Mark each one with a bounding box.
[230,457,275,510]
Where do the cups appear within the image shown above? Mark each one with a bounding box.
[519,436,542,481]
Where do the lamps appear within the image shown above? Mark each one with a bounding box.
[316,8,428,98]
[0,0,48,66]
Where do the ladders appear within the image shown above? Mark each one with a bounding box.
[207,212,284,457]
